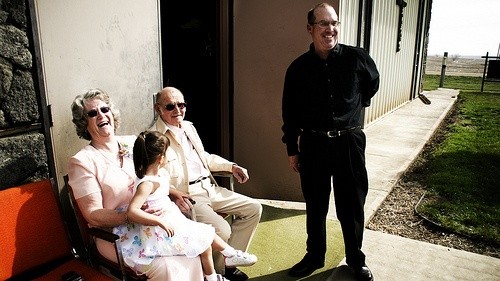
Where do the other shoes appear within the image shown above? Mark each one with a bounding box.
[205,273,231,281]
[224,250,258,269]
[222,266,248,281]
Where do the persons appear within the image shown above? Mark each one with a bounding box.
[69,90,204,281]
[128,131,257,281]
[148,87,262,281]
[282,3,379,281]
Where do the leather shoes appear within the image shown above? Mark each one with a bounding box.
[288,253,325,277]
[346,262,373,281]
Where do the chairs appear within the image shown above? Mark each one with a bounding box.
[63,174,146,281]
[0,178,120,281]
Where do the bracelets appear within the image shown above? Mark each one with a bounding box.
[126,215,128,223]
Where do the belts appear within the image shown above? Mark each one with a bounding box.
[303,125,362,138]
[189,176,211,185]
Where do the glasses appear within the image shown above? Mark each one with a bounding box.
[314,20,341,29]
[85,106,110,118]
[160,103,187,111]
